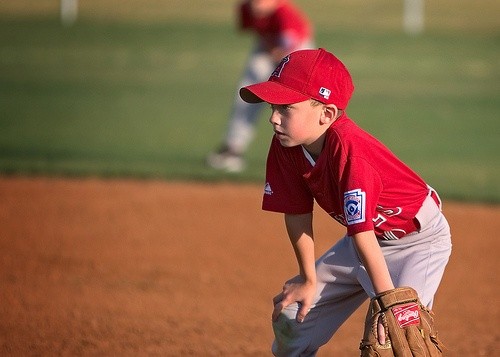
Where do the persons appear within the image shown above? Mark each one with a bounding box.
[240,48,454,357]
[204,0,318,172]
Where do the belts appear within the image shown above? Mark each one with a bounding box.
[377,190,439,240]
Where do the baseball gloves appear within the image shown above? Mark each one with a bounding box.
[360,287,447,357]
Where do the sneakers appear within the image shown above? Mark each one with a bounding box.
[206,152,243,173]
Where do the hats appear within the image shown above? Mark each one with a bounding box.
[239,47,354,111]
